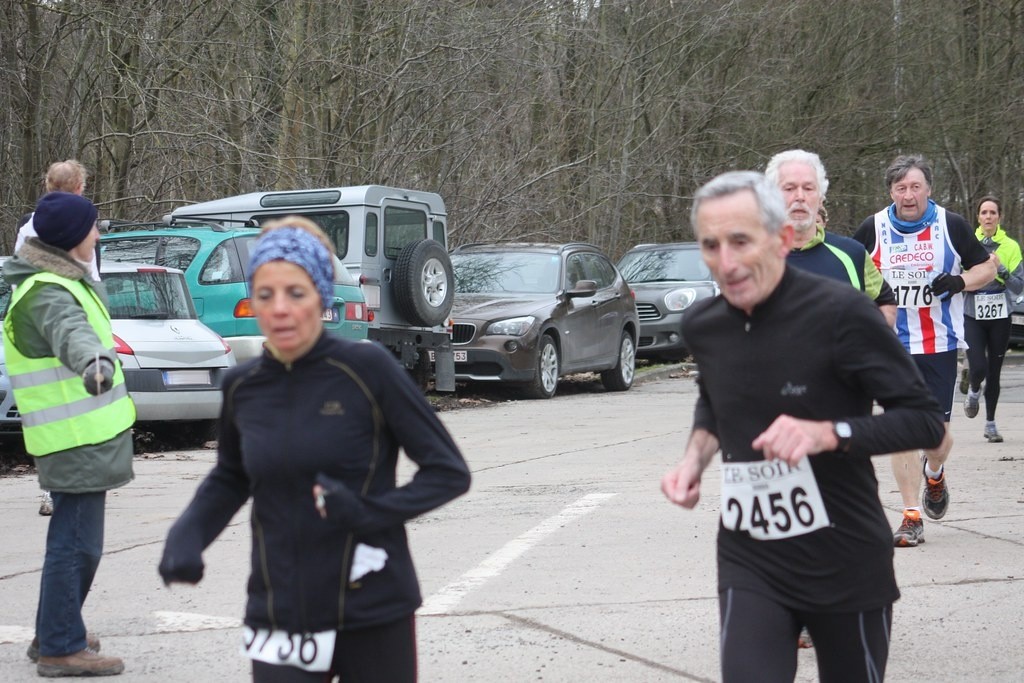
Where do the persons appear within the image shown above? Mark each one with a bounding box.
[851,156,995,547]
[156,217,469,683]
[815,205,828,229]
[0,192,135,677]
[661,171,944,683]
[10,158,101,517]
[958,196,1024,444]
[765,149,900,648]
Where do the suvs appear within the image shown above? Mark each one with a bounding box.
[90,218,369,365]
[162,184,456,390]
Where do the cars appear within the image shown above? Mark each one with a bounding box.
[615,244,720,364]
[0,256,236,464]
[413,239,639,400]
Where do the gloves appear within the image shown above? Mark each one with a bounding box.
[927,272,965,302]
[83,357,115,396]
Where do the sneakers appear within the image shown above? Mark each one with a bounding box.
[921,455,949,519]
[892,510,925,546]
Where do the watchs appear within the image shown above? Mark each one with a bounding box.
[832,419,852,452]
[1001,272,1009,279]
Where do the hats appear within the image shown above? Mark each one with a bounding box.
[34,192,98,252]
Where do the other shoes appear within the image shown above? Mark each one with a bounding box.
[964,386,980,418]
[798,629,813,649]
[960,368,969,394]
[27,635,102,660]
[38,492,53,514]
[38,646,125,677]
[984,421,1003,442]
